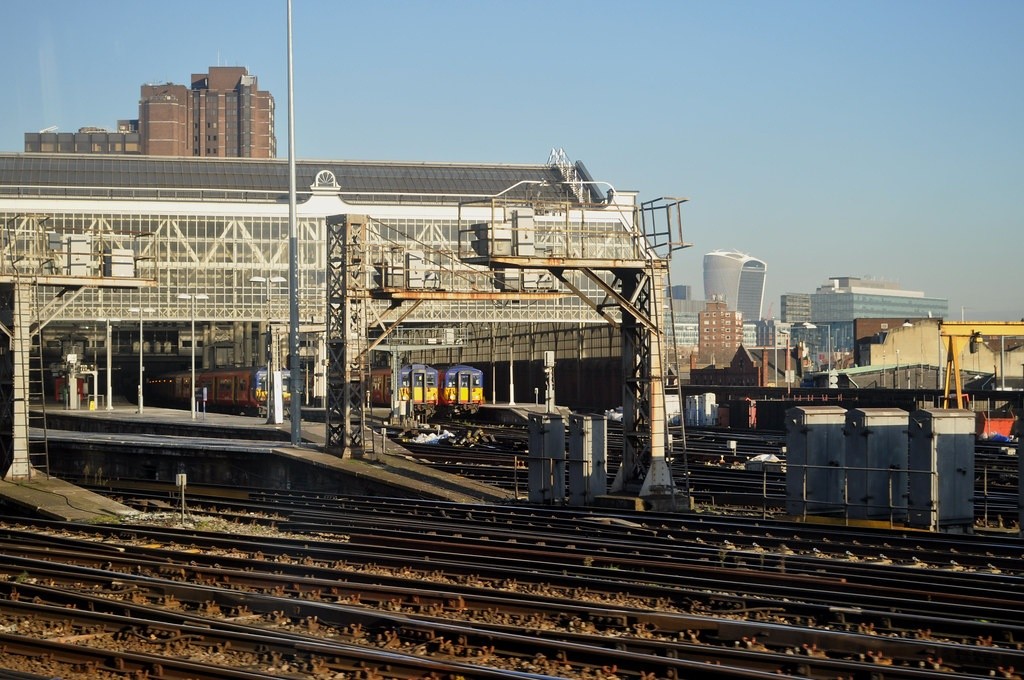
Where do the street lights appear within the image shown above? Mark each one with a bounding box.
[803,323,830,388]
[250,276,285,417]
[178,293,209,421]
[96,318,120,410]
[776,325,817,386]
[128,307,155,412]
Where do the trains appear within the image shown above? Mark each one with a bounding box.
[304,363,440,419]
[144,365,304,420]
[431,364,485,419]
